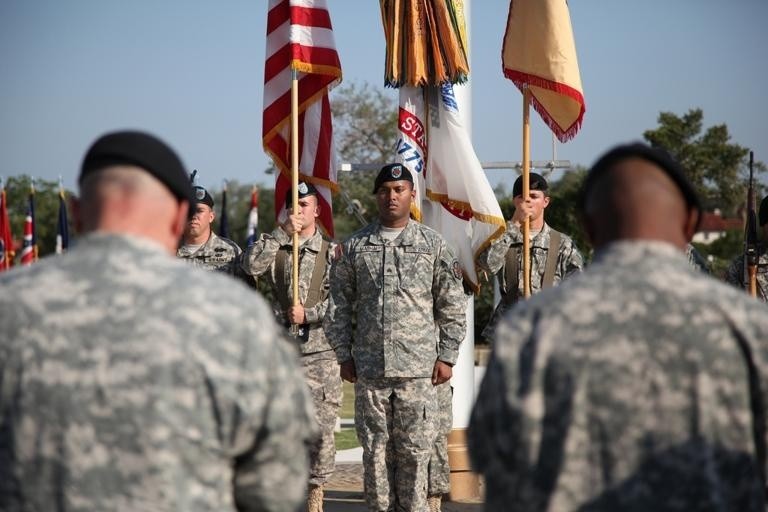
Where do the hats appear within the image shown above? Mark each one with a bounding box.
[513,174,548,196]
[575,144,702,235]
[193,187,215,206]
[285,183,315,209]
[79,131,194,209]
[373,164,413,193]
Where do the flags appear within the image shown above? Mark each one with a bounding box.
[381,1,507,293]
[220,187,230,235]
[17,184,37,272]
[499,0,587,141]
[0,189,19,272]
[56,181,70,258]
[260,0,344,240]
[244,187,265,247]
[730,189,765,294]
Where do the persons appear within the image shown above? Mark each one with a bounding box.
[481,172,583,304]
[325,168,466,510]
[242,181,339,510]
[471,147,766,512]
[2,131,311,510]
[178,188,243,278]
[424,376,453,512]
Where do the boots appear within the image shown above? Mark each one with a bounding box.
[428,494,442,512]
[308,485,323,512]
